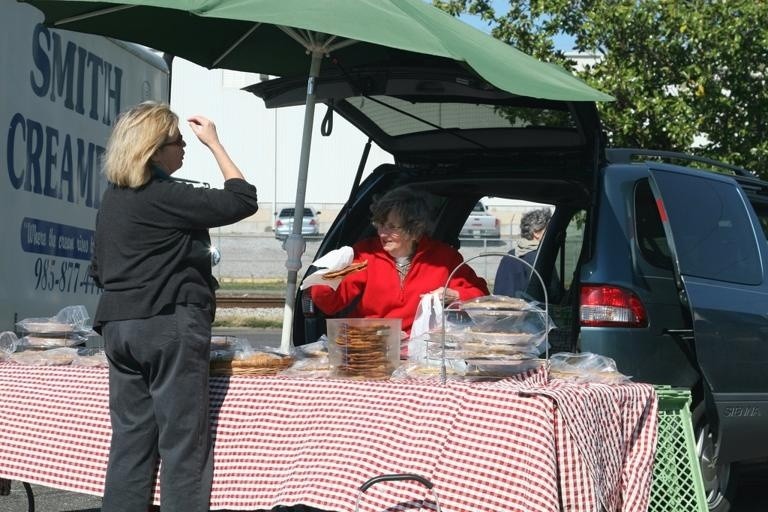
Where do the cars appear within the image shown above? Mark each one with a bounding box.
[458,201,502,241]
[274,206,322,242]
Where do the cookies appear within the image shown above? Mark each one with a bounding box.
[322,260,367,280]
[334,323,394,377]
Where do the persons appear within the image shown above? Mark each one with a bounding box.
[493,208,563,305]
[89,100,258,512]
[311,185,490,360]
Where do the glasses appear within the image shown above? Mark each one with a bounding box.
[162,132,183,147]
[373,220,406,233]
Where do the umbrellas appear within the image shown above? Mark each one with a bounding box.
[19,0,618,354]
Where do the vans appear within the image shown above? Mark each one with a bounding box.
[241,65,766,506]
